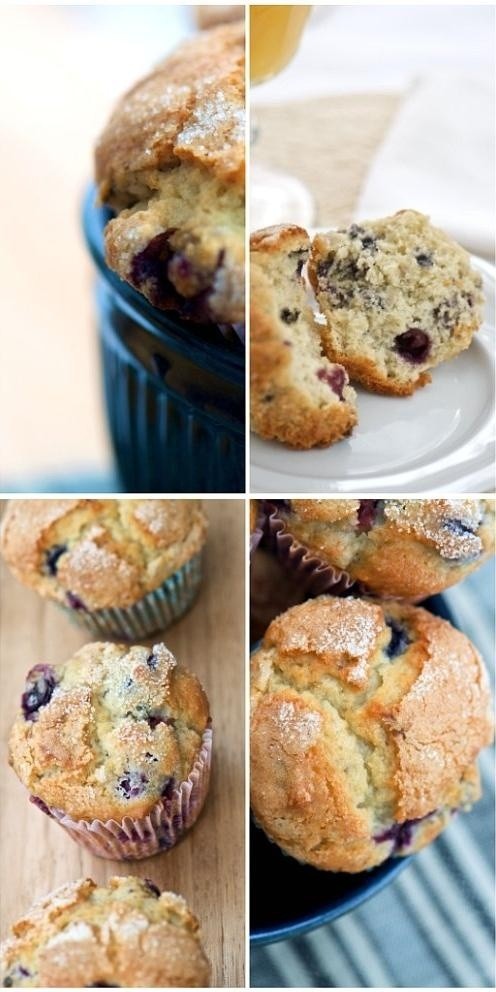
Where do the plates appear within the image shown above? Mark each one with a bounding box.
[249,223,495,497]
[244,512,475,955]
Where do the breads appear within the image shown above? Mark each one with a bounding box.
[93,18,486,450]
[1,500,209,609]
[281,499,496,596]
[9,638,211,819]
[3,877,213,988]
[250,595,495,874]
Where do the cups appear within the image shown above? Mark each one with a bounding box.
[76,98,244,489]
